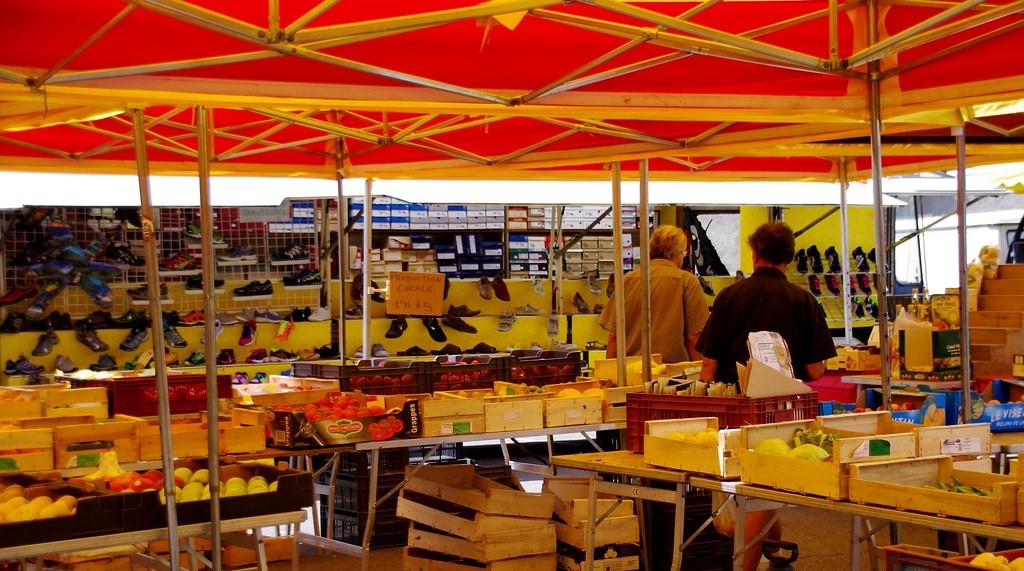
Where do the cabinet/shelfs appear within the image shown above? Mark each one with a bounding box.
[1,198,326,319]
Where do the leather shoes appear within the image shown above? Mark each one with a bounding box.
[345,273,616,358]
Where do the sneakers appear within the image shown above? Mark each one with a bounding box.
[736,271,745,282]
[0,205,336,385]
[795,245,896,322]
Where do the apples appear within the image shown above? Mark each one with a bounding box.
[853,403,914,413]
[0,484,77,524]
[118,467,278,504]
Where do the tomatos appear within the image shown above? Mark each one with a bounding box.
[272,396,385,422]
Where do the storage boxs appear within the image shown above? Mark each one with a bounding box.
[1,196,1024,571]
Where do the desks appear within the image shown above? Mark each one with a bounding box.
[0,507,307,571]
[689,473,1024,571]
[172,445,354,543]
[0,459,163,478]
[548,449,694,570]
[354,422,617,557]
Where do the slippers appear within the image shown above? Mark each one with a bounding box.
[697,277,715,296]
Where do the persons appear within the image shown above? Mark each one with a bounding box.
[694,221,837,571]
[598,225,709,363]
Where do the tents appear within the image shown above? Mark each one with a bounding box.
[1,0,1022,571]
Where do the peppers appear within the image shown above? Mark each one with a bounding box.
[920,475,992,496]
